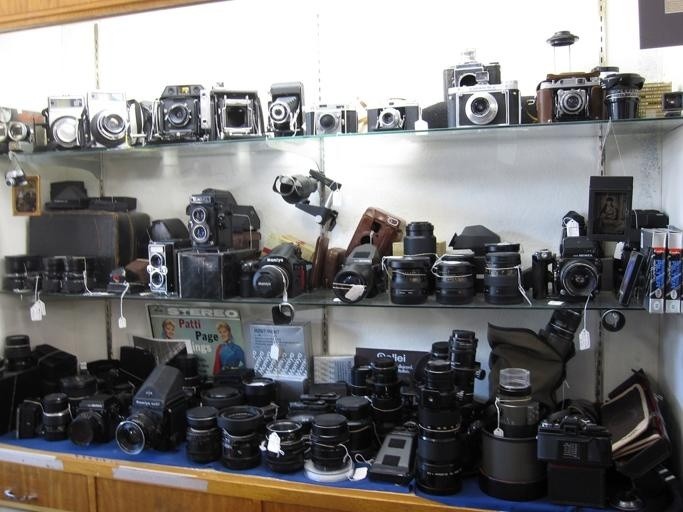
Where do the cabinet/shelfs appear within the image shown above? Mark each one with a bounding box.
[0,1,683,512]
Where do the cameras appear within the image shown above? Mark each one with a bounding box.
[0,47,683,512]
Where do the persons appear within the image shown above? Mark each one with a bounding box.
[213,322,246,373]
[160,319,176,339]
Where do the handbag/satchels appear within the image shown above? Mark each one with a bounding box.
[601,368,673,476]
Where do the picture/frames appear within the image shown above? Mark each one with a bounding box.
[586,176,633,242]
[11,175,41,216]
[638,0,683,49]
